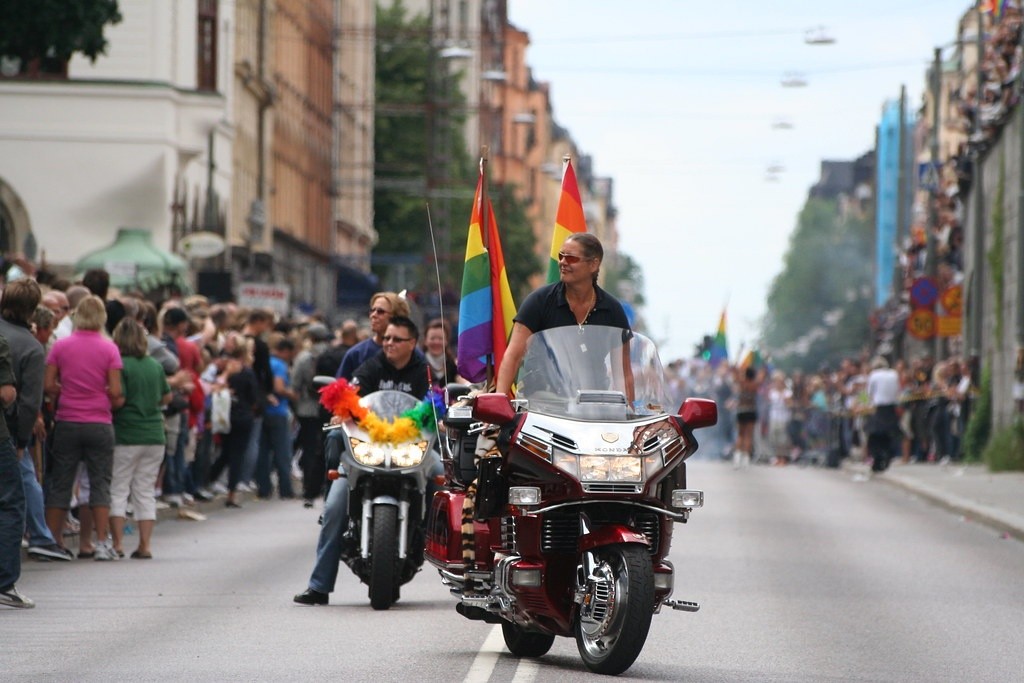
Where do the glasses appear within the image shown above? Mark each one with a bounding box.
[380,335,413,342]
[559,252,592,264]
[369,306,394,315]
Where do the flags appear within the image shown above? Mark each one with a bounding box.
[735,348,773,386]
[705,312,729,366]
[544,156,588,285]
[456,155,520,405]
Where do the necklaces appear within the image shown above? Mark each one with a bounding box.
[564,284,595,335]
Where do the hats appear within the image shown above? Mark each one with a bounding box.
[163,308,193,325]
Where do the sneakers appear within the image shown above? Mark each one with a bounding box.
[0,585,35,607]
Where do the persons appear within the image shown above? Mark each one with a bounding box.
[632,356,975,473]
[1,268,456,608]
[481,233,637,437]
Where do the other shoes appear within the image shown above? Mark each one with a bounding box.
[155,500,169,510]
[94,538,124,561]
[77,551,95,558]
[304,499,313,507]
[131,551,152,558]
[211,481,228,495]
[189,491,207,500]
[236,482,250,492]
[248,480,256,490]
[27,544,74,561]
[294,588,329,604]
[226,501,241,509]
[732,450,750,471]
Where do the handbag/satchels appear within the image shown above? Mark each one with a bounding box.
[211,385,230,434]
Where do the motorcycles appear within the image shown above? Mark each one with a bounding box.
[312,373,475,611]
[419,325,718,679]
